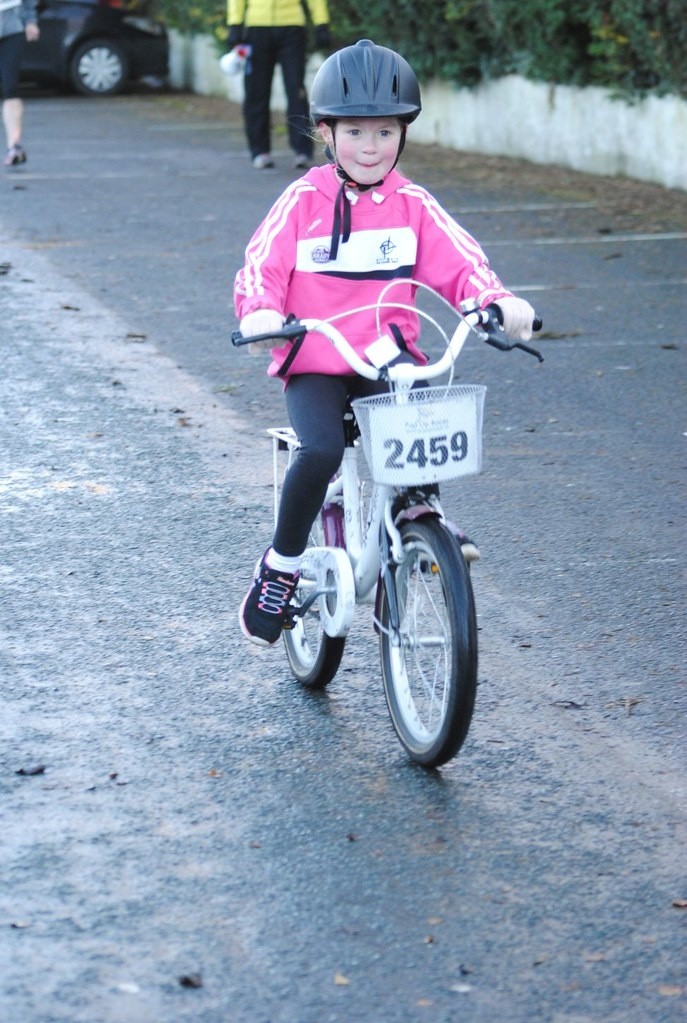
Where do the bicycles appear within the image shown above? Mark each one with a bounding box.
[229,279,546,770]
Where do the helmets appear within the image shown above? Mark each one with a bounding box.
[310,39,422,191]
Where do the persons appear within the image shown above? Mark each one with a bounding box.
[221,0,334,173]
[0,1,38,165]
[227,30,542,649]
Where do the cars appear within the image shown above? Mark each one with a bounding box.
[17,2,170,100]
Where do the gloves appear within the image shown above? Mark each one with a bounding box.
[314,24,330,48]
[239,308,288,356]
[494,296,536,342]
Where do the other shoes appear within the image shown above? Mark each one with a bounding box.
[4,145,26,165]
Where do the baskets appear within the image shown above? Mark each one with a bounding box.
[350,384,488,488]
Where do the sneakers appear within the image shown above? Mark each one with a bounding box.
[291,153,311,170]
[411,518,480,574]
[238,545,302,647]
[252,152,274,169]
[228,24,242,48]
